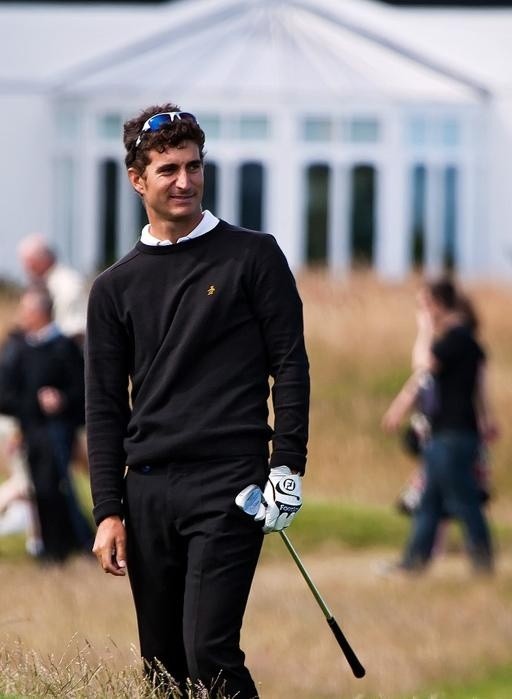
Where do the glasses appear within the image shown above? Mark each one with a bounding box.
[135,112,196,148]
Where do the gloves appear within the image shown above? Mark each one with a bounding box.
[253,465,302,534]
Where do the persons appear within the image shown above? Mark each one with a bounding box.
[1,287,97,568]
[378,278,505,578]
[84,104,311,699]
[1,232,91,512]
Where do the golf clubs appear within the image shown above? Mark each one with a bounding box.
[235,486,366,684]
